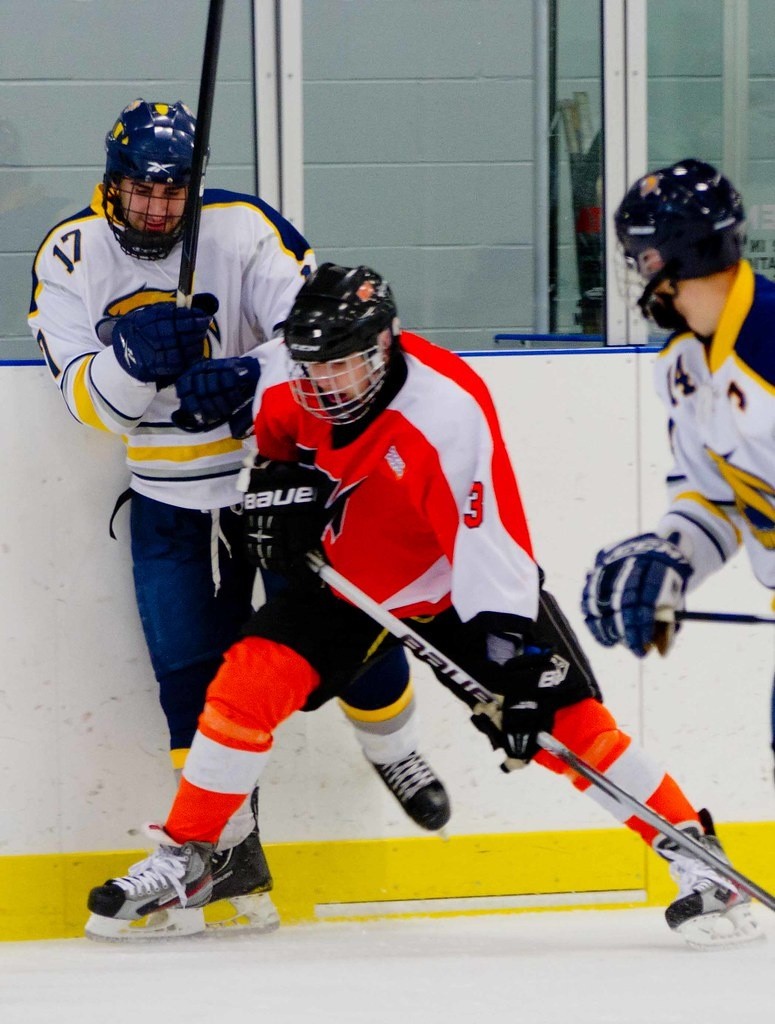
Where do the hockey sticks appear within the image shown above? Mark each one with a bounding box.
[174,0,224,313]
[671,605,775,628]
[306,547,775,917]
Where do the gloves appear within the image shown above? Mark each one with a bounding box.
[111,293,220,384]
[580,531,695,657]
[171,356,261,434]
[247,515,305,579]
[470,655,555,778]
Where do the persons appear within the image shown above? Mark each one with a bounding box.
[577,161,775,782]
[27,98,452,943]
[87,261,764,955]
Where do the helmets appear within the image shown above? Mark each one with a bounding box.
[282,262,398,429]
[614,158,747,330]
[103,98,210,262]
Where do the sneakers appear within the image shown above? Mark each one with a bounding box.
[657,806,775,946]
[203,824,281,937]
[84,839,216,941]
[367,752,452,835]
